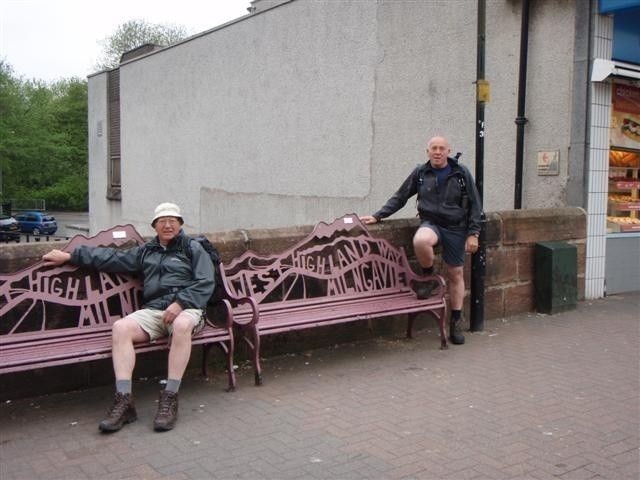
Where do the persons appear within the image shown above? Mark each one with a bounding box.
[40,200,219,434]
[355,134,484,346]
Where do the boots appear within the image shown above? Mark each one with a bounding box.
[450,317,464,344]
[417,268,434,299]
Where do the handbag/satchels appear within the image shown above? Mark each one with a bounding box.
[186,234,228,306]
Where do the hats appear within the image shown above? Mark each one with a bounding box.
[150,203,184,227]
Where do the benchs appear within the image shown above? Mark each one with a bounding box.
[217,213,451,385]
[0,224,237,392]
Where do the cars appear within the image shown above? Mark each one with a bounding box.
[0,211,58,241]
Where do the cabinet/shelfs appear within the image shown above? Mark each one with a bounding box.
[607,167,640,233]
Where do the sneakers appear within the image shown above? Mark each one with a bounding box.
[153,388,178,430]
[99,392,137,430]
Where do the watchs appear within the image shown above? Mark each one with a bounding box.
[471,232,480,238]
